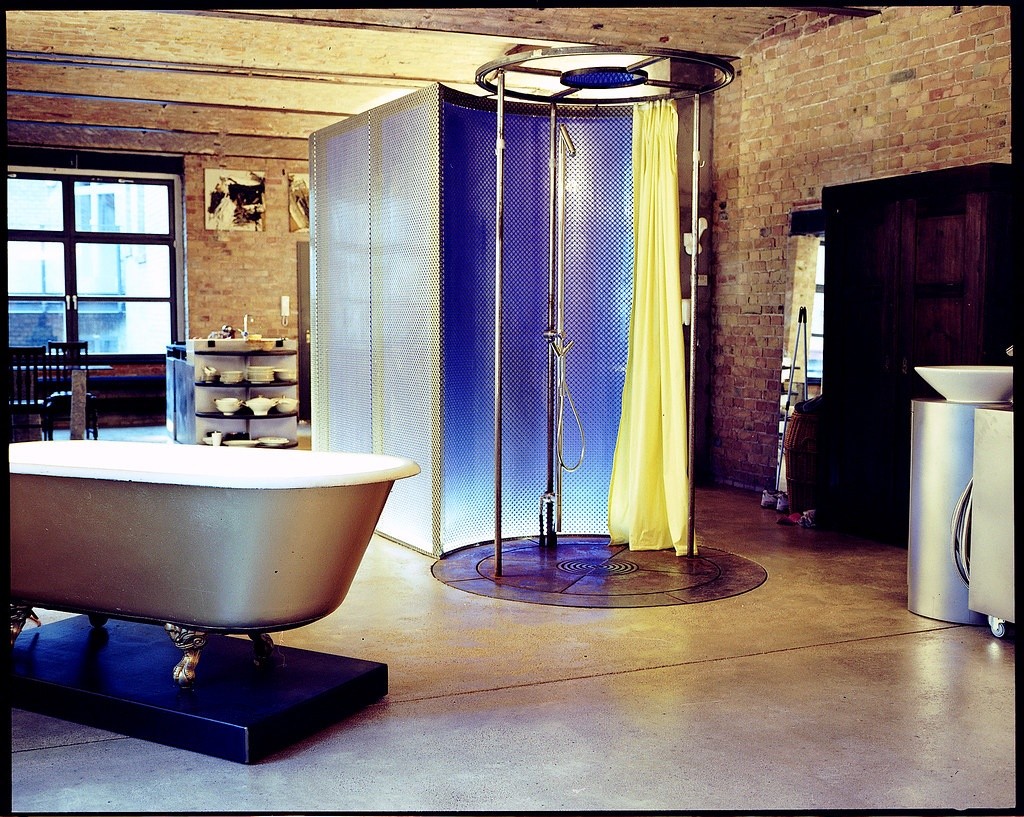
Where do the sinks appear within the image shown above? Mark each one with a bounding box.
[912,365,1013,405]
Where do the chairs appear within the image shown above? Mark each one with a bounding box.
[8,345,51,443]
[46,340,98,440]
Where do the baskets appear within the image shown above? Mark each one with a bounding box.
[783,408,817,516]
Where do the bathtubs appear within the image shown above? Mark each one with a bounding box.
[9,442,422,694]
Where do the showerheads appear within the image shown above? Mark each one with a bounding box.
[559,122,578,158]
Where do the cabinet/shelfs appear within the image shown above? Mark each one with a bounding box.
[816,163,1012,550]
[166,355,195,446]
[194,350,299,450]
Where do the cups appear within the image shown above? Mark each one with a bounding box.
[250,335,262,339]
[212,432,221,445]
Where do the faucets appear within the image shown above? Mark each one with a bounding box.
[1005,345,1013,357]
[238,313,253,340]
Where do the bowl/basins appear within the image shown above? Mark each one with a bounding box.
[202,438,216,444]
[273,370,293,380]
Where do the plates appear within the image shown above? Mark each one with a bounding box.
[223,440,258,447]
[259,437,289,446]
[219,365,274,384]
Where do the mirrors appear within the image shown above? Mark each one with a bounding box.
[775,228,825,496]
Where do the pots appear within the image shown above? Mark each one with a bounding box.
[213,398,241,415]
[241,395,279,417]
[275,396,298,412]
